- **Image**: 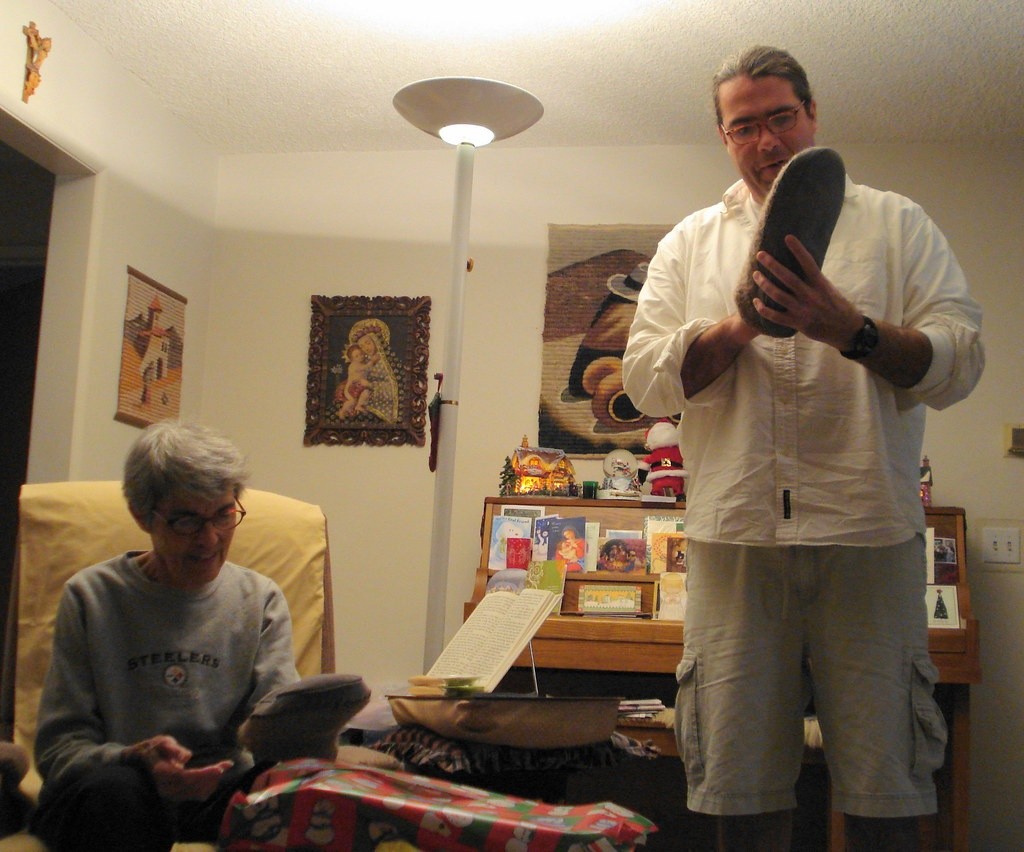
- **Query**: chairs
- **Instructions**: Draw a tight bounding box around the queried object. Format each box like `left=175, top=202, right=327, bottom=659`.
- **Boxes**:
left=0, top=482, right=334, bottom=851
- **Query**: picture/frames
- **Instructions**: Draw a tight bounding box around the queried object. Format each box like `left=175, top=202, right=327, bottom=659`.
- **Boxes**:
left=304, top=296, right=432, bottom=448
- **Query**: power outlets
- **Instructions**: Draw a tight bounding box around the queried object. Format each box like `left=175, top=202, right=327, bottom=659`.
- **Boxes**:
left=983, top=526, right=1019, bottom=563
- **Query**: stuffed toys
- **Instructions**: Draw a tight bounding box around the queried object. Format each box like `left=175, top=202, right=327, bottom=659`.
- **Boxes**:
left=638, top=418, right=688, bottom=501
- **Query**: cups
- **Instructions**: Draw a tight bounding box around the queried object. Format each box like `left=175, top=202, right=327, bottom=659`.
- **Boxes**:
left=583, top=481, right=598, bottom=499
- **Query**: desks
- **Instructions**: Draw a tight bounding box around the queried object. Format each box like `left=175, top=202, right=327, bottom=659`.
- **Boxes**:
left=371, top=694, right=626, bottom=807
left=465, top=498, right=980, bottom=851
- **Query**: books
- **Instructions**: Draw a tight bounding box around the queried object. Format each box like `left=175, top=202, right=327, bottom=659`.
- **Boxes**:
left=423, top=588, right=564, bottom=694
left=488, top=503, right=648, bottom=575
left=640, top=516, right=691, bottom=620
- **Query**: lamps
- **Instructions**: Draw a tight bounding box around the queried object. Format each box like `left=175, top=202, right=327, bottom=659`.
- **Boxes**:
left=393, top=77, right=544, bottom=678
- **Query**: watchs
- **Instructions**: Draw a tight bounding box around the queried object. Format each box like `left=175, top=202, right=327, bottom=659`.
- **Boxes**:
left=840, top=315, right=880, bottom=360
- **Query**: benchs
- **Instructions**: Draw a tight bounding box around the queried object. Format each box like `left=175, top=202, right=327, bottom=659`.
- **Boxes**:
left=617, top=709, right=847, bottom=852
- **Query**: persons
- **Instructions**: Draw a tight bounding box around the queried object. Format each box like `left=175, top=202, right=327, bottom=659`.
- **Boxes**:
left=622, top=41, right=988, bottom=852
left=32, top=422, right=302, bottom=852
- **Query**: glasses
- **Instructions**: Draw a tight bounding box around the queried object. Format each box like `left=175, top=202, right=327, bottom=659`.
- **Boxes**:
left=718, top=99, right=805, bottom=145
left=146, top=492, right=245, bottom=534
